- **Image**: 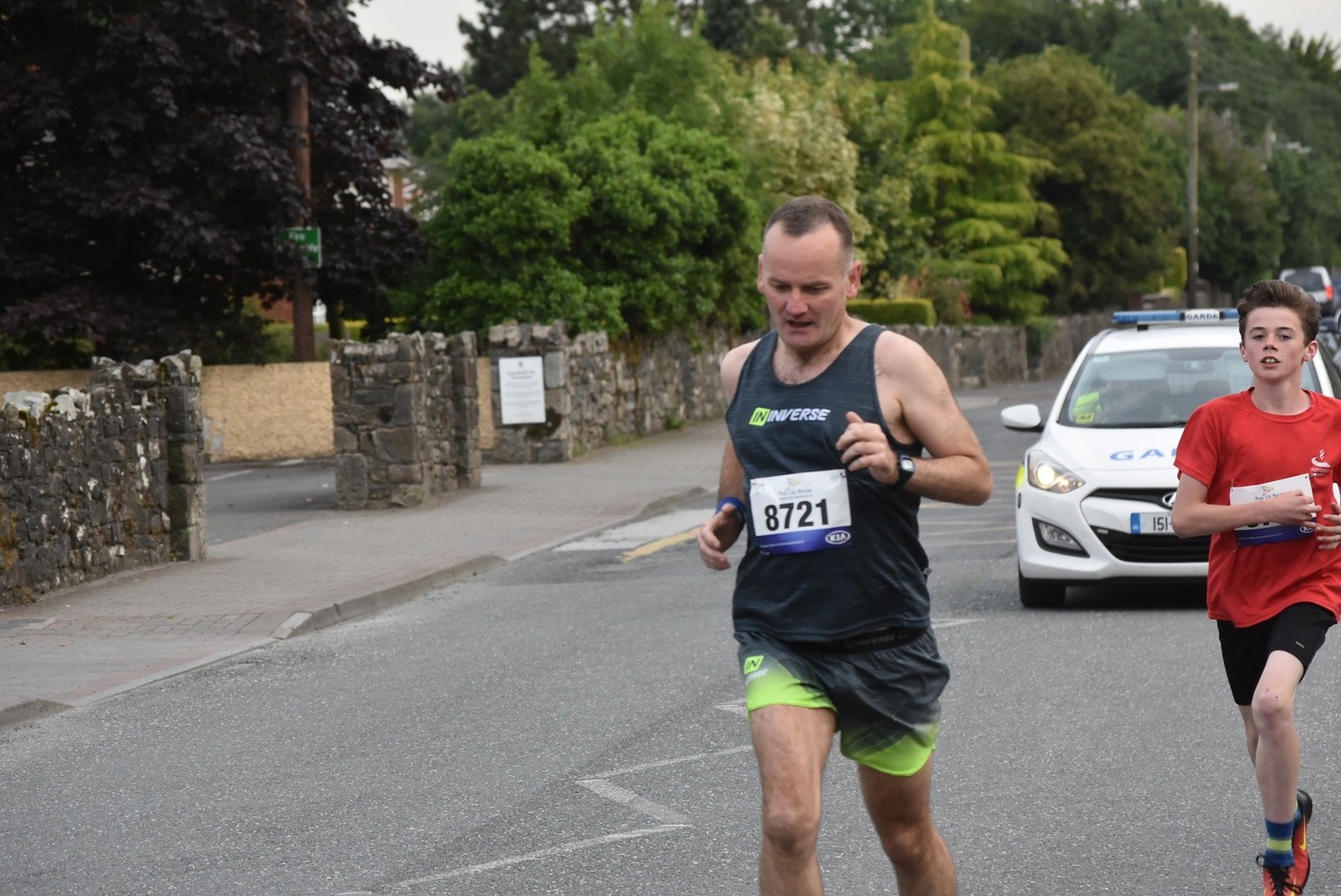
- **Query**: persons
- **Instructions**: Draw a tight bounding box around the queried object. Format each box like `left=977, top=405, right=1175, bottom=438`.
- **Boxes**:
left=696, top=194, right=991, bottom=895
left=1172, top=279, right=1341, bottom=896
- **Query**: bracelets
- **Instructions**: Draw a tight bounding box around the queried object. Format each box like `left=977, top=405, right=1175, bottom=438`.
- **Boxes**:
left=714, top=496, right=746, bottom=524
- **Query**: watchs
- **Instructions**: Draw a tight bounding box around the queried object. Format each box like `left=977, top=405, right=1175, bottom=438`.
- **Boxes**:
left=894, top=453, right=915, bottom=488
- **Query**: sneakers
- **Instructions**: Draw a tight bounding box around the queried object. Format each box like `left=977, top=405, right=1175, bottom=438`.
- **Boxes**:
left=1255, top=854, right=1297, bottom=896
left=1291, top=790, right=1313, bottom=891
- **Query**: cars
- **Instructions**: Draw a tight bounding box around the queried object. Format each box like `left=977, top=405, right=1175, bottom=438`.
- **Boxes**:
left=1280, top=265, right=1337, bottom=314
left=998, top=308, right=1340, bottom=610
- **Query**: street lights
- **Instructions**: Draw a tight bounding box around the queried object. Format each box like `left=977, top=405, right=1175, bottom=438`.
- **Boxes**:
left=1185, top=82, right=1238, bottom=306
